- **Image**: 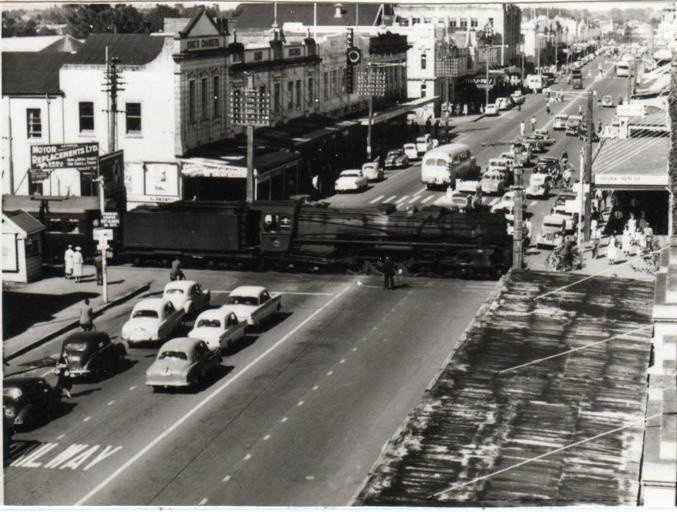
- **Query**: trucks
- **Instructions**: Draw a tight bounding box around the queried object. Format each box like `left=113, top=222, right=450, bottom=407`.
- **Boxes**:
left=571, top=69, right=582, bottom=88
left=445, top=179, right=482, bottom=208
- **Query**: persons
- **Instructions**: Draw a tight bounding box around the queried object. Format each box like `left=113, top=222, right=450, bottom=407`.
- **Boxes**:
left=94, top=252, right=105, bottom=284
left=63, top=244, right=75, bottom=280
left=519, top=82, right=659, bottom=273
left=78, top=298, right=98, bottom=332
left=70, top=246, right=85, bottom=283
left=2, top=343, right=10, bottom=379
left=382, top=256, right=397, bottom=291
left=398, top=115, right=449, bottom=144
left=55, top=350, right=74, bottom=398
left=169, top=257, right=185, bottom=281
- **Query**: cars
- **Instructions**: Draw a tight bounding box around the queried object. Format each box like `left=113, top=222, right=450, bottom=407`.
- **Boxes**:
left=334, top=162, right=384, bottom=193
left=3, top=376, right=63, bottom=430
left=481, top=129, right=560, bottom=222
left=56, top=330, right=126, bottom=383
left=536, top=190, right=578, bottom=248
left=602, top=94, right=613, bottom=107
left=188, top=308, right=247, bottom=352
left=482, top=90, right=524, bottom=115
left=163, top=280, right=209, bottom=314
left=220, top=285, right=281, bottom=326
left=553, top=114, right=599, bottom=142
left=146, top=337, right=222, bottom=393
left=616, top=62, right=630, bottom=78
left=385, top=136, right=433, bottom=168
left=121, top=298, right=184, bottom=348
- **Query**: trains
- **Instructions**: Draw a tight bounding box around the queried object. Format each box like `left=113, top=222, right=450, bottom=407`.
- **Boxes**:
left=2, top=193, right=512, bottom=282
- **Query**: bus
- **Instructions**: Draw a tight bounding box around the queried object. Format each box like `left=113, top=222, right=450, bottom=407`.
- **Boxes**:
left=421, top=143, right=476, bottom=189
